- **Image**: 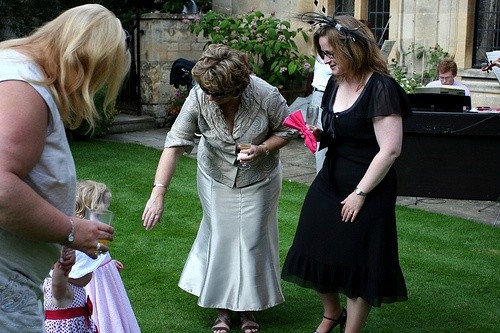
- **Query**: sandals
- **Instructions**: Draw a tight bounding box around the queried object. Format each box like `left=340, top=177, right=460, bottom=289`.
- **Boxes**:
left=239, top=310, right=261, bottom=333
left=212, top=316, right=232, bottom=333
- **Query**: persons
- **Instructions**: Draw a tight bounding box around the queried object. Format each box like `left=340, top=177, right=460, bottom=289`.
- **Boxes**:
left=312, top=20, right=372, bottom=173
left=142, top=43, right=299, bottom=333
left=495, top=58, right=500, bottom=68
left=281, top=6, right=413, bottom=333
left=0, top=4, right=143, bottom=333
left=424, top=59, right=472, bottom=107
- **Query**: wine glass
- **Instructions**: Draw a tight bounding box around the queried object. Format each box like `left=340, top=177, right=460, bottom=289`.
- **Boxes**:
left=237, top=126, right=253, bottom=171
left=303, top=106, right=319, bottom=146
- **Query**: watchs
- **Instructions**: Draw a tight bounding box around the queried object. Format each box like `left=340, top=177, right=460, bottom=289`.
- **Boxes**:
left=353, top=186, right=367, bottom=196
left=59, top=216, right=75, bottom=245
left=262, top=143, right=270, bottom=156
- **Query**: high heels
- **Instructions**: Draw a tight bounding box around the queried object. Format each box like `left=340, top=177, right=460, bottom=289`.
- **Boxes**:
left=313, top=307, right=347, bottom=333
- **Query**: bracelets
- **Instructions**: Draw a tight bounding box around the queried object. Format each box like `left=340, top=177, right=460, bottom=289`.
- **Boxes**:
left=153, top=184, right=168, bottom=190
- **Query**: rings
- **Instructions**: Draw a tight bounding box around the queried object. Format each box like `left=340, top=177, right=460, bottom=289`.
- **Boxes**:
left=96, top=242, right=101, bottom=250
left=349, top=211, right=352, bottom=214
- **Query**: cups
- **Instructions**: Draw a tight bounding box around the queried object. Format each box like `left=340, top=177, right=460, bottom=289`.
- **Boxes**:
left=85, top=209, right=114, bottom=256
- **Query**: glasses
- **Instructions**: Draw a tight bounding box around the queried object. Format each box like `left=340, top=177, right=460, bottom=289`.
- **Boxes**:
left=198, top=82, right=233, bottom=102
left=319, top=47, right=343, bottom=60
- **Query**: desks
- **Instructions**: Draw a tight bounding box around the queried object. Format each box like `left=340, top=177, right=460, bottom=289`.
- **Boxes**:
left=396, top=109, right=500, bottom=202
